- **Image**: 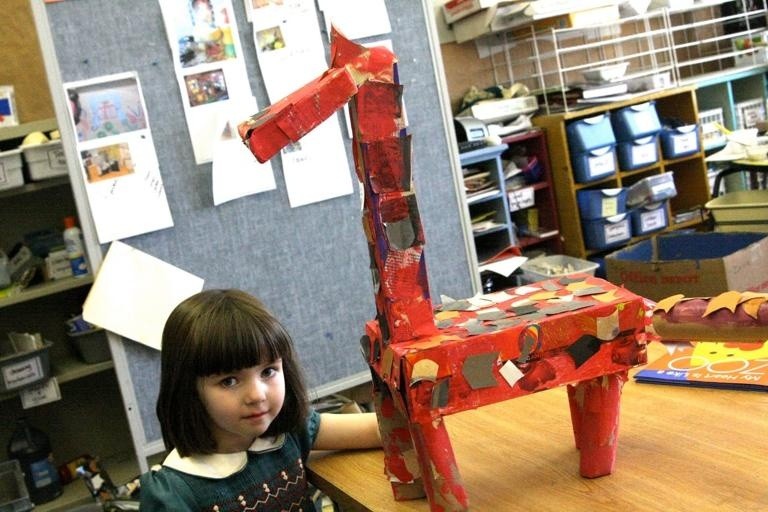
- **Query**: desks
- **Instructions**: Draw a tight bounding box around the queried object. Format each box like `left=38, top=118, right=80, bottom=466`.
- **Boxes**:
left=307, top=337, right=767, bottom=512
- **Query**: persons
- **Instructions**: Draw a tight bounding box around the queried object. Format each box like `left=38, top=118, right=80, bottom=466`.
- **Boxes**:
left=140, top=289, right=386, bottom=511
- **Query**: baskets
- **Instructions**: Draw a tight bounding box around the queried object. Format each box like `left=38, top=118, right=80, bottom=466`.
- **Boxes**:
left=698, top=107, right=726, bottom=147
left=734, top=99, right=764, bottom=129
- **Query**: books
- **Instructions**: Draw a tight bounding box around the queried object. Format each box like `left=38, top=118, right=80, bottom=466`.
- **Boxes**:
left=634, top=340, right=768, bottom=392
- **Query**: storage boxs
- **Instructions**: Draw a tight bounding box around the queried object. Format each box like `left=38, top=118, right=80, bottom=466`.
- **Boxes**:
left=0, top=148, right=24, bottom=192
left=603, top=232, right=767, bottom=301
left=0, top=339, right=53, bottom=397
left=19, top=138, right=68, bottom=181
left=67, top=328, right=112, bottom=364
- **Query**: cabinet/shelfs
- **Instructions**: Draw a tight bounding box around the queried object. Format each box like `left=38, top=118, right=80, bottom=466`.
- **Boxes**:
left=0, top=116, right=142, bottom=512
